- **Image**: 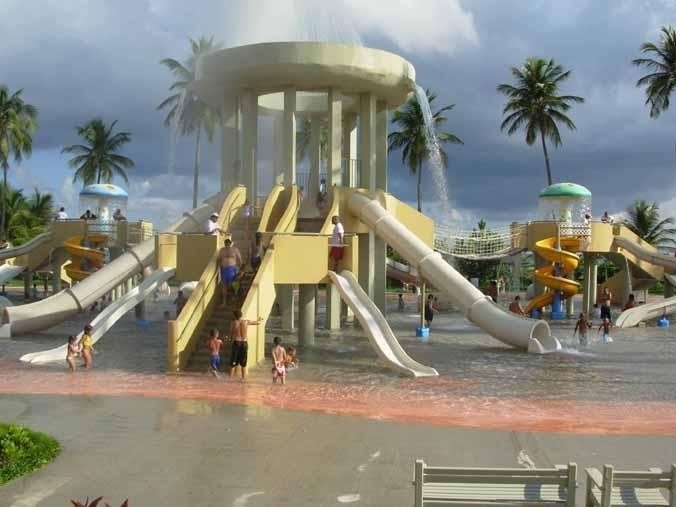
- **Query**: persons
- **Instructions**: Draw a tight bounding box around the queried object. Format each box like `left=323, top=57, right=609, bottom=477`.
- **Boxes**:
left=572, top=310, right=595, bottom=348
left=433, top=296, right=442, bottom=312
left=595, top=316, right=616, bottom=344
left=296, top=183, right=304, bottom=209
left=200, top=209, right=231, bottom=236
left=250, top=229, right=269, bottom=272
left=327, top=213, right=345, bottom=272
left=227, top=309, right=264, bottom=380
left=113, top=208, right=128, bottom=220
left=398, top=292, right=406, bottom=312
left=0, top=238, right=9, bottom=250
left=625, top=292, right=637, bottom=309
left=171, top=289, right=188, bottom=317
left=509, top=294, right=525, bottom=315
left=285, top=346, right=298, bottom=370
left=481, top=278, right=498, bottom=303
left=29, top=282, right=42, bottom=300
left=601, top=211, right=615, bottom=223
left=78, top=209, right=97, bottom=219
left=314, top=174, right=337, bottom=221
left=202, top=326, right=227, bottom=382
left=237, top=199, right=255, bottom=232
left=54, top=206, right=68, bottom=219
left=425, top=293, right=441, bottom=327
left=269, top=335, right=290, bottom=385
left=598, top=285, right=617, bottom=328
left=218, top=238, right=242, bottom=307
left=78, top=324, right=97, bottom=367
left=65, top=333, right=81, bottom=372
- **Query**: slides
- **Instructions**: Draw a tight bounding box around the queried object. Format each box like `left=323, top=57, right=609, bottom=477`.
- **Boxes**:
left=348, top=193, right=562, bottom=354
left=20, top=268, right=177, bottom=363
left=0, top=194, right=224, bottom=338
left=523, top=236, right=582, bottom=313
left=329, top=269, right=440, bottom=378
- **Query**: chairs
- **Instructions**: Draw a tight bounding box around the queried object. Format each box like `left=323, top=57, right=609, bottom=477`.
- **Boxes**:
left=584, top=463, right=676, bottom=506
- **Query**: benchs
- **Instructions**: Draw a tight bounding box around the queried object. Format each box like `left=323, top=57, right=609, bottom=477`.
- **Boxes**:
left=411, top=457, right=580, bottom=506
